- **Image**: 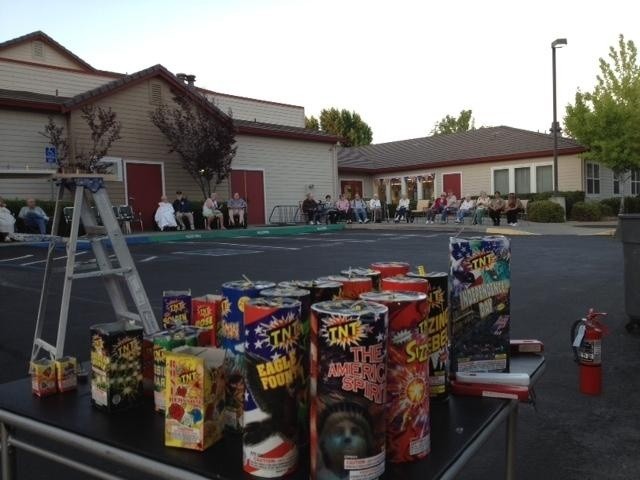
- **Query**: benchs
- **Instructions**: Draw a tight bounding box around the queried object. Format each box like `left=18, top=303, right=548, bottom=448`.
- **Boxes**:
left=64, top=206, right=143, bottom=234
left=299, top=201, right=390, bottom=223
left=446, top=200, right=528, bottom=226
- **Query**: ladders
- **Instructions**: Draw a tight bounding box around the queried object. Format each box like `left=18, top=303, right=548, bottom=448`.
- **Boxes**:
left=28, top=174, right=161, bottom=375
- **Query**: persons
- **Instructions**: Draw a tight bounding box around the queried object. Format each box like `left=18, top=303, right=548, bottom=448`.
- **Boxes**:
left=0, top=199, right=16, bottom=242
left=173, top=191, right=195, bottom=231
left=18, top=198, right=50, bottom=234
left=202, top=193, right=227, bottom=230
left=302, top=190, right=525, bottom=227
left=154, top=196, right=177, bottom=231
left=227, top=192, right=247, bottom=226
left=316, top=401, right=374, bottom=480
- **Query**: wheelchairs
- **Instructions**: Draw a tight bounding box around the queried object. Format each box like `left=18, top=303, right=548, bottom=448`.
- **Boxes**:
left=395, top=203, right=414, bottom=224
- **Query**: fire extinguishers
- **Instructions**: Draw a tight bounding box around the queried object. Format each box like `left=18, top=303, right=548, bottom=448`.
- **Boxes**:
left=570, top=308, right=610, bottom=395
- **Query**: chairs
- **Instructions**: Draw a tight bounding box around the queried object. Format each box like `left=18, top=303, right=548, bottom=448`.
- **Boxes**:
left=204, top=216, right=220, bottom=229
left=411, top=199, right=431, bottom=223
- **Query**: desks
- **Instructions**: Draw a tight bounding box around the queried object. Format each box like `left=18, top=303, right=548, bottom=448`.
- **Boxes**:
left=0, top=352, right=546, bottom=480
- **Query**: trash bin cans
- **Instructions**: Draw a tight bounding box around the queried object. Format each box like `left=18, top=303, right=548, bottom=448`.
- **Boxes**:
left=617, top=213, right=640, bottom=334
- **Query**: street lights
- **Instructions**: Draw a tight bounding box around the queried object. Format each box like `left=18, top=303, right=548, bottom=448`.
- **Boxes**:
left=549, top=36, right=568, bottom=194
